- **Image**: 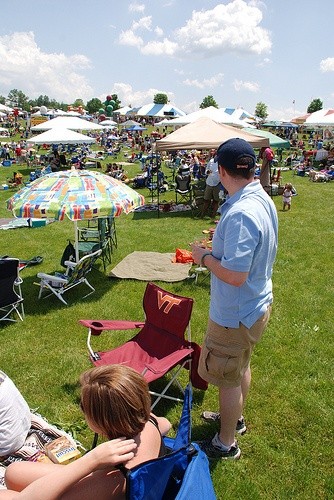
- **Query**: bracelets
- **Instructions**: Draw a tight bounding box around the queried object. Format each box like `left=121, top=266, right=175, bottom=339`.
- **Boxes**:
left=201, top=253, right=211, bottom=264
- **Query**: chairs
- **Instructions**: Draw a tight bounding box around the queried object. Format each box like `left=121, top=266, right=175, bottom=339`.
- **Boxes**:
left=0, top=215, right=217, bottom=500
left=146, top=166, right=195, bottom=204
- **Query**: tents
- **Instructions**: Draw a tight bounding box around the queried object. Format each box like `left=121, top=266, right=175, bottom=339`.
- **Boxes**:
left=26, top=127, right=98, bottom=169
left=99, top=103, right=257, bottom=131
left=31, top=116, right=114, bottom=130
left=152, top=119, right=290, bottom=217
left=0, top=104, right=81, bottom=121
left=261, top=108, right=334, bottom=131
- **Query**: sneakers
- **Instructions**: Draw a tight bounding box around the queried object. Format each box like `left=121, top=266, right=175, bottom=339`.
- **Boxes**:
left=202, top=411, right=246, bottom=434
left=192, top=432, right=241, bottom=461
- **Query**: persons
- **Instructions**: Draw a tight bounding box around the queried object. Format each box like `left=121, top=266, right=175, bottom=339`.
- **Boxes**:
left=190, top=138, right=278, bottom=466
left=0, top=363, right=171, bottom=500
left=191, top=147, right=292, bottom=220
left=0, top=116, right=195, bottom=192
left=277, top=126, right=334, bottom=179
left=12, top=436, right=138, bottom=500
left=0, top=370, right=31, bottom=462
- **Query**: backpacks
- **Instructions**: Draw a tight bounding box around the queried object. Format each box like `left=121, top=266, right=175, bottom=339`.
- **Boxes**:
left=61, top=241, right=76, bottom=268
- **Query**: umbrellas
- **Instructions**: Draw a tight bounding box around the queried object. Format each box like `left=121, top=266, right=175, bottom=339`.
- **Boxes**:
left=7, top=166, right=144, bottom=263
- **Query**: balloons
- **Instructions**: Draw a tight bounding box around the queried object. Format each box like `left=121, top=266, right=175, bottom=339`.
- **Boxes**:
left=99, top=94, right=120, bottom=121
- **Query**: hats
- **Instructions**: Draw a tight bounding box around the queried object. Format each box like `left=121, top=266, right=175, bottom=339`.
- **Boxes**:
left=212, top=137, right=255, bottom=173
